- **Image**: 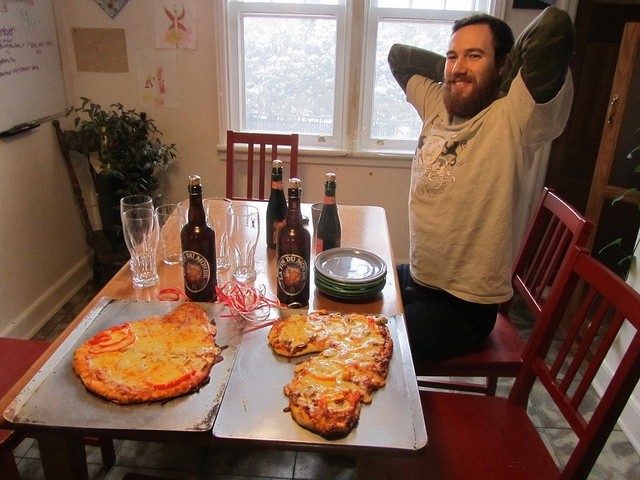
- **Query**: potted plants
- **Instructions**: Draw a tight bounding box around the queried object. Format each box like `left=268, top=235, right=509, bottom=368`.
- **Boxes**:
left=66, top=99, right=175, bottom=249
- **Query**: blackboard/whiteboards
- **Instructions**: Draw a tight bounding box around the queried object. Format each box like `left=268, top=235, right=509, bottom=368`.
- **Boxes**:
left=1, top=0, right=70, bottom=138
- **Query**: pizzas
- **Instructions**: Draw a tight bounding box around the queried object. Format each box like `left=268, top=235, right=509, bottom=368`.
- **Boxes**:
left=267, top=309, right=394, bottom=440
left=72, top=302, right=229, bottom=406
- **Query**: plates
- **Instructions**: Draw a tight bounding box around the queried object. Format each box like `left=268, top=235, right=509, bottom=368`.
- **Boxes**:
left=313, top=247, right=387, bottom=299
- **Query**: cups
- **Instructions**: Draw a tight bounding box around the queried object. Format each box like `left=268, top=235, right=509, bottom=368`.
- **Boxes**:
left=227, top=205, right=260, bottom=281
left=122, top=208, right=160, bottom=288
left=177, top=199, right=209, bottom=233
left=121, top=195, right=154, bottom=271
left=156, top=204, right=188, bottom=265
left=202, top=197, right=234, bottom=273
left=311, top=202, right=338, bottom=233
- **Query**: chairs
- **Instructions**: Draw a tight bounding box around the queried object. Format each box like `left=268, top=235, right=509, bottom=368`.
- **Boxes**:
left=52, top=120, right=131, bottom=288
left=356, top=249, right=640, bottom=480
left=228, top=130, right=297, bottom=201
left=414, top=185, right=594, bottom=397
left=0, top=338, right=117, bottom=480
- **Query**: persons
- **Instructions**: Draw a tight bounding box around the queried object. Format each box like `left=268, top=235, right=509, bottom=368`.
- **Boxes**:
left=388, top=9, right=573, bottom=367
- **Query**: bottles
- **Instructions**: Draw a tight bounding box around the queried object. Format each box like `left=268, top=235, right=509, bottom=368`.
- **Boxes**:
left=277, top=178, right=310, bottom=307
left=181, top=175, right=217, bottom=302
left=267, top=160, right=288, bottom=250
left=316, top=173, right=340, bottom=255
